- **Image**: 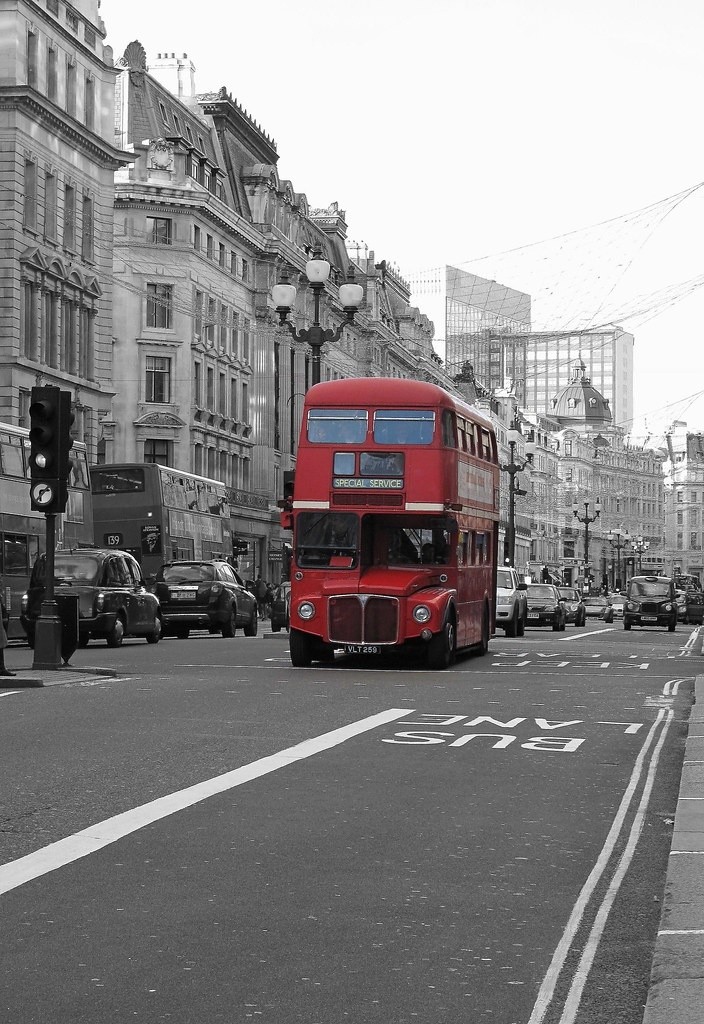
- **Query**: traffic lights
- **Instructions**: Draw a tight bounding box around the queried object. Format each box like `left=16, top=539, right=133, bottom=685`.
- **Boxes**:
left=29, top=387, right=61, bottom=512
left=283, top=470, right=294, bottom=529
left=232, top=536, right=248, bottom=568
left=60, top=391, right=75, bottom=513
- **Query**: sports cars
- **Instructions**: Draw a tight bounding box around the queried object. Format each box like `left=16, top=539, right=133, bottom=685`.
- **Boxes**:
left=583, top=597, right=614, bottom=623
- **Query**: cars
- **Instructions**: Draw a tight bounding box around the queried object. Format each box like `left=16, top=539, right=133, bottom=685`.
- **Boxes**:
left=557, top=586, right=587, bottom=627
left=675, top=591, right=691, bottom=623
left=621, top=575, right=681, bottom=631
left=526, top=583, right=567, bottom=631
left=610, top=596, right=626, bottom=617
left=23, top=548, right=165, bottom=648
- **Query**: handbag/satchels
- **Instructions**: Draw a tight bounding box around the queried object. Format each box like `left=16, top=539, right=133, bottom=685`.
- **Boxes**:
left=258, top=583, right=268, bottom=598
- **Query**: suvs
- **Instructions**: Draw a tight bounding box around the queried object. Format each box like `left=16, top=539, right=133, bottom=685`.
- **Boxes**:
left=495, top=567, right=528, bottom=637
left=270, top=582, right=292, bottom=629
left=152, top=559, right=259, bottom=638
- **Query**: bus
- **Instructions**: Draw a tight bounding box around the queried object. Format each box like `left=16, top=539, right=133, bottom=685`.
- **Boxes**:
left=88, top=463, right=234, bottom=592
left=0, top=422, right=95, bottom=640
left=278, top=376, right=498, bottom=665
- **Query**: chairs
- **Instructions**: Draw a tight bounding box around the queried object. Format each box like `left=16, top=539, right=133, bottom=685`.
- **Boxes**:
left=421, top=543, right=435, bottom=564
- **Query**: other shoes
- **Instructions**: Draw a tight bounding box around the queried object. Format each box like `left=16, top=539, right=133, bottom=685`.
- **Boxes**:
left=0, top=670, right=16, bottom=676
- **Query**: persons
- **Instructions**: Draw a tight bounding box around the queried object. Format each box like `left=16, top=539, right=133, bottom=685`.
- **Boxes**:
left=330, top=521, right=356, bottom=556
left=419, top=543, right=444, bottom=564
left=256, top=576, right=279, bottom=621
left=0, top=605, right=15, bottom=676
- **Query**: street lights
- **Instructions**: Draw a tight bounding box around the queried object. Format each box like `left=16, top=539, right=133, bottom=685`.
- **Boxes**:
left=267, top=243, right=366, bottom=386
left=631, top=534, right=651, bottom=575
left=606, top=523, right=631, bottom=592
left=573, top=491, right=601, bottom=596
left=498, top=420, right=537, bottom=567
